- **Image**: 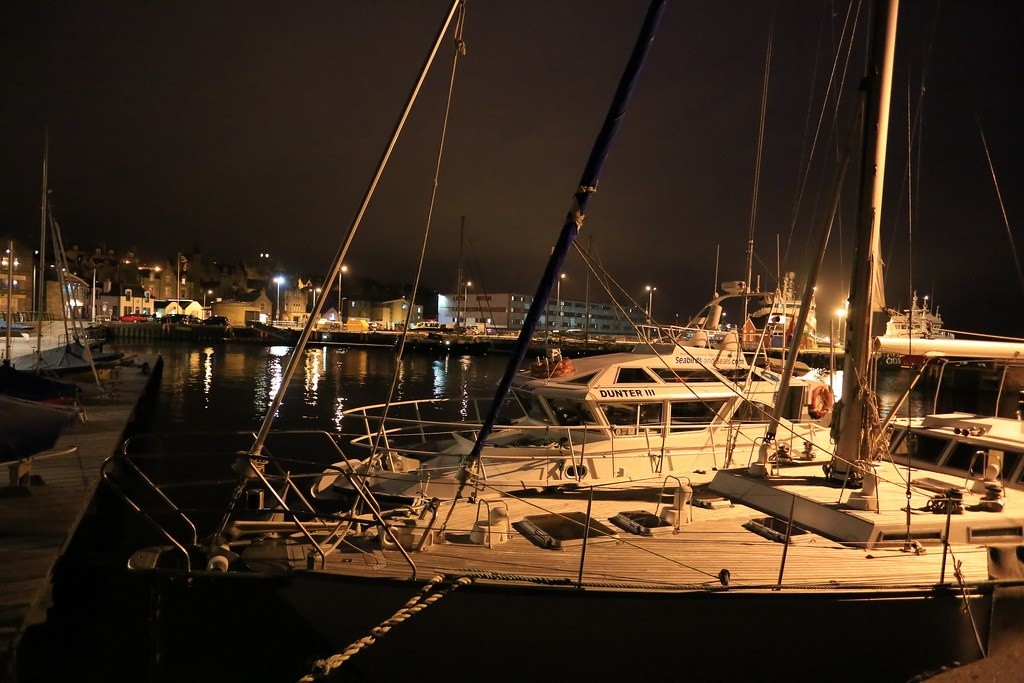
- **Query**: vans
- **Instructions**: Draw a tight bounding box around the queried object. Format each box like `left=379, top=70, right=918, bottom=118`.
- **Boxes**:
left=412, top=322, right=437, bottom=333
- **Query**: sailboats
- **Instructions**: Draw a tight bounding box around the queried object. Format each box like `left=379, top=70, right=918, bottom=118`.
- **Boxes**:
left=0, top=134, right=123, bottom=395
left=96, top=1, right=1023, bottom=659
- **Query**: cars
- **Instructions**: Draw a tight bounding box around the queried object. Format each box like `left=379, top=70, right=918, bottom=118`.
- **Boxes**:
left=486, top=329, right=502, bottom=336
left=201, top=316, right=230, bottom=327
left=118, top=314, right=147, bottom=324
left=157, top=314, right=189, bottom=324
left=472, top=329, right=481, bottom=335
left=187, top=314, right=203, bottom=324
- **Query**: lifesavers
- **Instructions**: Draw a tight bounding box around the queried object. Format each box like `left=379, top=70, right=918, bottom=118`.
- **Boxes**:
left=530, top=355, right=571, bottom=378
left=807, top=387, right=833, bottom=419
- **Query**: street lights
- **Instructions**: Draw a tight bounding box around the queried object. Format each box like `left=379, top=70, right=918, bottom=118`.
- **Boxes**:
left=313, top=288, right=321, bottom=307
left=338, top=266, right=347, bottom=315
left=276, top=277, right=284, bottom=321
left=464, top=281, right=472, bottom=327
left=557, top=273, right=566, bottom=320
left=646, top=285, right=657, bottom=343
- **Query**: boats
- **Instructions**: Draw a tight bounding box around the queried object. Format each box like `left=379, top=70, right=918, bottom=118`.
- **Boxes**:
left=0, top=397, right=78, bottom=468
left=881, top=291, right=954, bottom=369
